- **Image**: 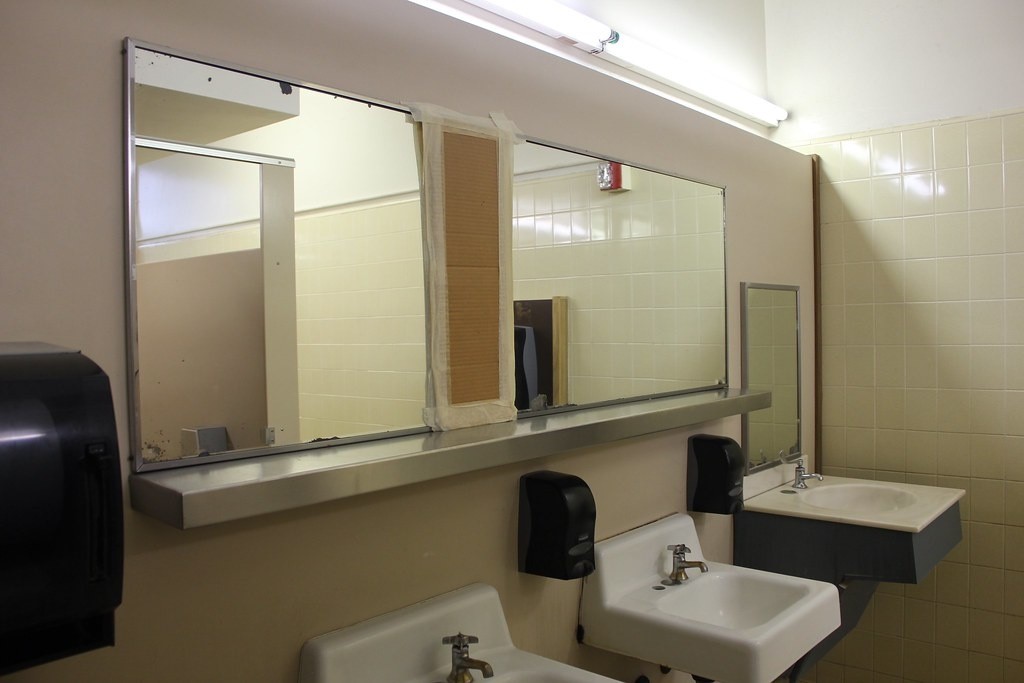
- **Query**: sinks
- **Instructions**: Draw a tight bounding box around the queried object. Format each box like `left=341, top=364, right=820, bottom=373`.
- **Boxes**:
left=296, top=582, right=620, bottom=683
left=803, top=485, right=916, bottom=513
left=579, top=513, right=842, bottom=683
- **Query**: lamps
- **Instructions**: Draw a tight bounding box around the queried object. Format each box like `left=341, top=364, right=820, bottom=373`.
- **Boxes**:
left=595, top=29, right=790, bottom=129
left=461, top=0, right=616, bottom=55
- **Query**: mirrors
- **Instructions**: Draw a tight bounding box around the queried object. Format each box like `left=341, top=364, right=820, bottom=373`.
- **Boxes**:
left=120, top=33, right=436, bottom=475
left=510, top=131, right=731, bottom=427
left=739, top=278, right=804, bottom=477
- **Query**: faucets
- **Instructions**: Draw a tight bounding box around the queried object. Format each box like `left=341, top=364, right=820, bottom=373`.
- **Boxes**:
left=666, top=543, right=708, bottom=579
left=758, top=448, right=767, bottom=464
left=440, top=633, right=494, bottom=683
left=779, top=452, right=824, bottom=489
